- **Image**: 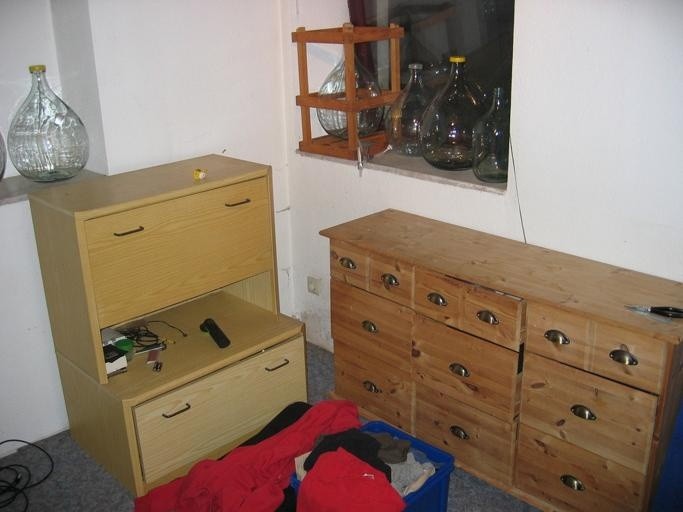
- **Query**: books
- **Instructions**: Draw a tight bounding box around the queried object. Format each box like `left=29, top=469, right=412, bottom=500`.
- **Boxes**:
left=107, top=367, right=127, bottom=379
left=102, top=344, right=127, bottom=373
left=100, top=327, right=124, bottom=347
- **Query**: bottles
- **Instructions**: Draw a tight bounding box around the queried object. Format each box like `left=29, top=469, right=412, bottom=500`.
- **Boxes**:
left=316, top=46, right=383, bottom=141
left=6, top=65, right=89, bottom=183
left=384, top=55, right=508, bottom=184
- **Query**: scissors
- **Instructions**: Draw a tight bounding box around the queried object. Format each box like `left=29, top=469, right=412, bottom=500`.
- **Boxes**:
left=624, top=305, right=683, bottom=318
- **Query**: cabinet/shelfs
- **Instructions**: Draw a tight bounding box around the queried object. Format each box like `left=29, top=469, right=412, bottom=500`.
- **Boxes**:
left=513, top=301, right=681, bottom=510
left=411, top=263, right=517, bottom=493
left=330, top=239, right=413, bottom=442
left=24, top=154, right=277, bottom=384
left=50, top=326, right=308, bottom=495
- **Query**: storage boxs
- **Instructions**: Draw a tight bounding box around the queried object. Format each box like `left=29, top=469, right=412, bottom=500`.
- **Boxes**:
left=289, top=422, right=455, bottom=510
